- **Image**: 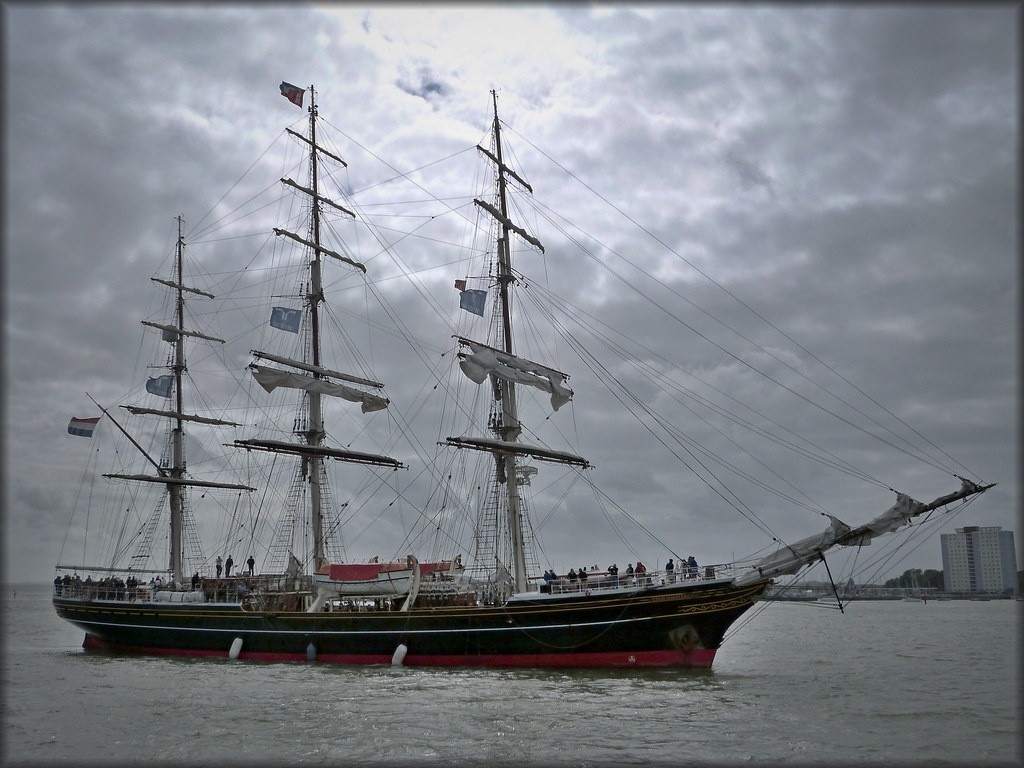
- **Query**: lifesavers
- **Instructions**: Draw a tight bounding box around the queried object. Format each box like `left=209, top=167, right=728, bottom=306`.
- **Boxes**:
left=585, top=590, right=590, bottom=597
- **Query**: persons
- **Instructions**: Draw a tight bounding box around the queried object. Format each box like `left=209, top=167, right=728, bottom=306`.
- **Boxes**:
left=216, top=556, right=223, bottom=578
left=54, top=575, right=139, bottom=603
left=225, top=555, right=233, bottom=578
left=247, top=555, right=255, bottom=577
left=367, top=598, right=381, bottom=611
left=543, top=555, right=700, bottom=591
left=192, top=572, right=199, bottom=591
left=149, top=575, right=166, bottom=587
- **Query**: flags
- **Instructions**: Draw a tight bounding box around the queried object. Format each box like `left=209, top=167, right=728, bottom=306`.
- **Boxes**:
left=454, top=279, right=488, bottom=317
left=279, top=80, right=306, bottom=109
left=146, top=375, right=174, bottom=397
left=269, top=306, right=303, bottom=334
left=68, top=416, right=100, bottom=438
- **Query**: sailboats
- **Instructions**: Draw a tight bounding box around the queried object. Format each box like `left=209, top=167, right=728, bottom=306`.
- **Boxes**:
left=51, top=77, right=998, bottom=669
